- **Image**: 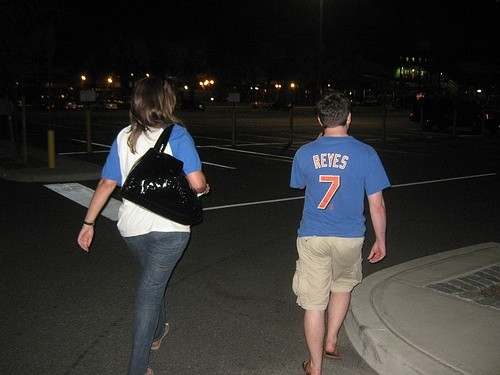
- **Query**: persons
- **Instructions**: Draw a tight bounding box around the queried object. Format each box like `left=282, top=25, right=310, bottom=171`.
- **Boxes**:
left=290, top=93, right=390, bottom=375
left=78, top=75, right=209, bottom=375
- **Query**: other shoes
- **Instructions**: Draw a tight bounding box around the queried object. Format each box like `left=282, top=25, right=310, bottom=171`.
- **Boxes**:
left=151, top=322, right=169, bottom=349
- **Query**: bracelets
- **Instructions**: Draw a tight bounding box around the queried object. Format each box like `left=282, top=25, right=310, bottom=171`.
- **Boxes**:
left=83, top=221, right=94, bottom=225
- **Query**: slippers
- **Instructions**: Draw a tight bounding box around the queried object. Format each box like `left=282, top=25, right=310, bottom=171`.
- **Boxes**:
left=323, top=349, right=340, bottom=359
left=303, top=360, right=311, bottom=375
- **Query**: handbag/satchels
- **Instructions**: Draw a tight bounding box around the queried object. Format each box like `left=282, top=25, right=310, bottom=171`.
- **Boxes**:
left=120, top=125, right=203, bottom=225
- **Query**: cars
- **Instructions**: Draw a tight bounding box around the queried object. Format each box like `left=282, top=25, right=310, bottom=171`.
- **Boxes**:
left=410, top=93, right=500, bottom=132
left=36, top=90, right=120, bottom=112
